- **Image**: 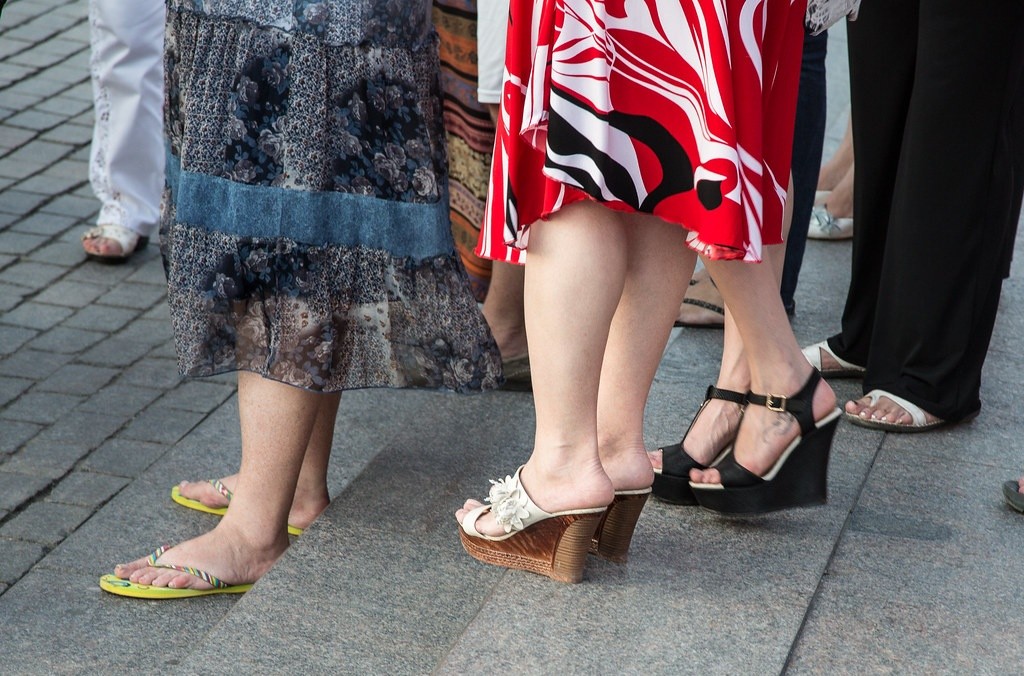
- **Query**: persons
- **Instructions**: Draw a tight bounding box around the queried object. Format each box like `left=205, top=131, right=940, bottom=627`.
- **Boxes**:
left=454, top=0, right=808, bottom=584
left=671, top=169, right=794, bottom=328
left=98, top=0, right=505, bottom=599
left=81, top=0, right=165, bottom=264
left=646, top=167, right=844, bottom=516
left=780, top=0, right=862, bottom=319
left=801, top=0, right=1023, bottom=512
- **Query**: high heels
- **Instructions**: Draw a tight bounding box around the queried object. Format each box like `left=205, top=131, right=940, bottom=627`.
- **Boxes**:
left=588, top=486, right=652, bottom=562
left=688, top=366, right=843, bottom=516
left=650, top=384, right=749, bottom=505
left=459, top=464, right=608, bottom=583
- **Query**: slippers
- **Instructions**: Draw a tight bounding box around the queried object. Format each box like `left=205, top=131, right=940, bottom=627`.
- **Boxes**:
left=99, top=545, right=254, bottom=599
left=171, top=479, right=304, bottom=535
left=496, top=352, right=533, bottom=393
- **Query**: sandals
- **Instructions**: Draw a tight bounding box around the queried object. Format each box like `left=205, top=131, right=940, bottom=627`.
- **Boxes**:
left=845, top=389, right=981, bottom=432
left=673, top=276, right=795, bottom=330
left=81, top=224, right=148, bottom=266
left=801, top=340, right=866, bottom=380
left=1002, top=481, right=1024, bottom=513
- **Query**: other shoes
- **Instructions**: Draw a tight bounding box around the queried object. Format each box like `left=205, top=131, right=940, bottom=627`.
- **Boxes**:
left=807, top=205, right=853, bottom=239
left=814, top=190, right=832, bottom=205
